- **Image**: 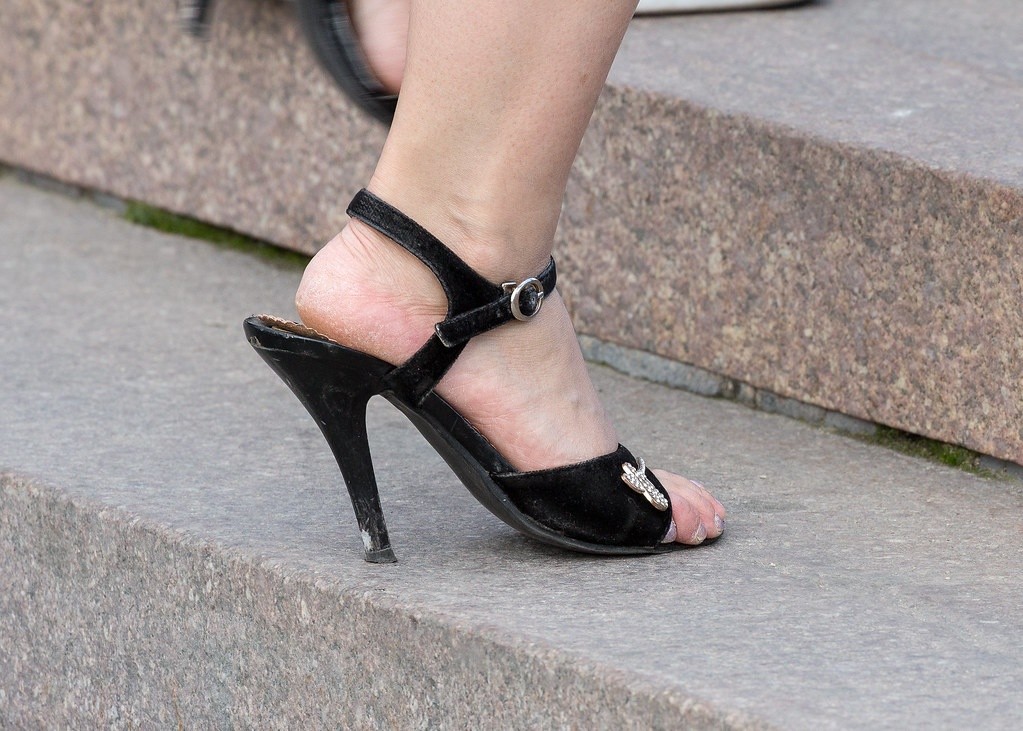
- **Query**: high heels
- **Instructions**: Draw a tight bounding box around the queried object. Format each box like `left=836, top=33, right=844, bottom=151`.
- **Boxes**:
left=187, top=0, right=401, bottom=127
left=243, top=190, right=720, bottom=566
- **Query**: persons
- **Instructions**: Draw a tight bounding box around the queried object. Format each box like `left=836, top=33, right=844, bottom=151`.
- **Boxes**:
left=241, top=0, right=726, bottom=566
